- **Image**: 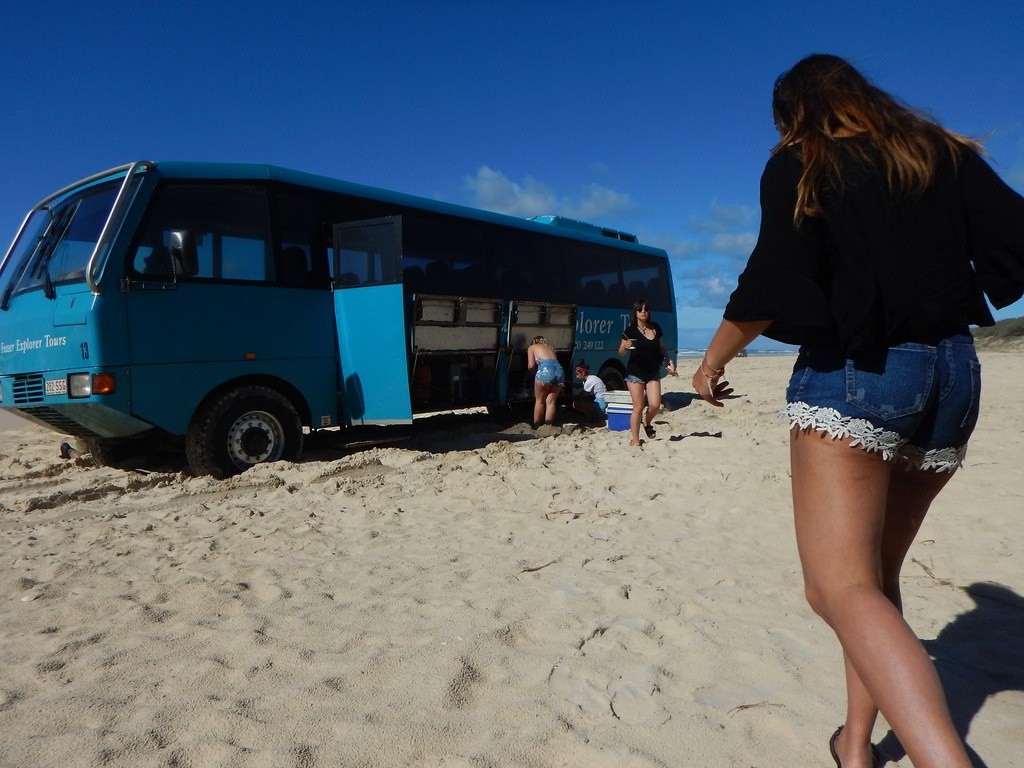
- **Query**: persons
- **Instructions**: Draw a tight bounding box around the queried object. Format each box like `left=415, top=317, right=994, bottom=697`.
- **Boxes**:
left=618, top=299, right=675, bottom=446
left=692, top=55, right=1024, bottom=768
left=570, top=363, right=607, bottom=424
left=528, top=335, right=565, bottom=424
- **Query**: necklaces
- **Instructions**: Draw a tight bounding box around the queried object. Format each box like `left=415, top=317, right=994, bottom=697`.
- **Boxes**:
left=638, top=324, right=649, bottom=334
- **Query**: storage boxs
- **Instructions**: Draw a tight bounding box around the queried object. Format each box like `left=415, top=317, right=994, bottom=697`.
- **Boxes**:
left=606, top=404, right=634, bottom=432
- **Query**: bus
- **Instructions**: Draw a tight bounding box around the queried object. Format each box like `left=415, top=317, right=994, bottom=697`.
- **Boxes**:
left=1, top=158, right=678, bottom=481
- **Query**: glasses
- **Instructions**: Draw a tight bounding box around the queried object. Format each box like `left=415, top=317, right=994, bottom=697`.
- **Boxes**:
left=635, top=307, right=649, bottom=313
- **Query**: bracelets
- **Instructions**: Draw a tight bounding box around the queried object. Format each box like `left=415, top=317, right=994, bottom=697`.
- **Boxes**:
left=700, top=351, right=725, bottom=398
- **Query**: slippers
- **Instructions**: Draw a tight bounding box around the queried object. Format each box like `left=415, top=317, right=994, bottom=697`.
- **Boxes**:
left=830, top=725, right=877, bottom=768
left=643, top=424, right=656, bottom=439
